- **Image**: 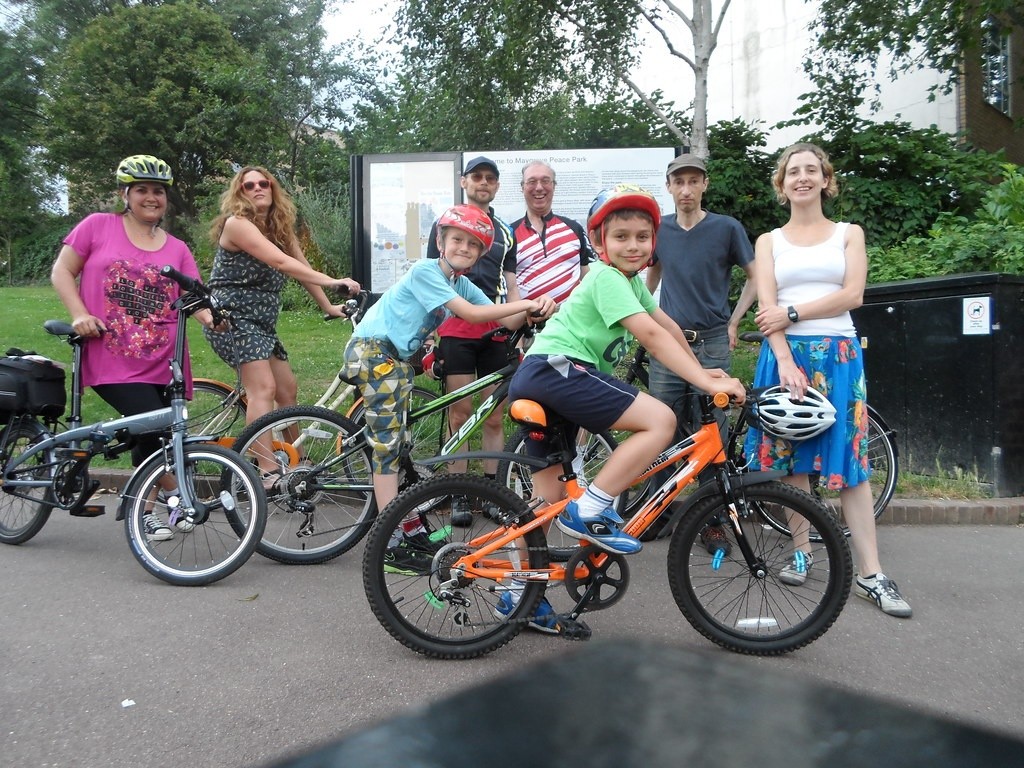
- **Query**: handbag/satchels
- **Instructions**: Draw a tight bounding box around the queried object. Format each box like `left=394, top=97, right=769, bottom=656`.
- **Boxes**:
left=0, top=348, right=67, bottom=414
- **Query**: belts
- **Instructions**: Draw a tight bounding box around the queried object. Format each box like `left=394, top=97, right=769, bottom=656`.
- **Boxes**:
left=681, top=325, right=727, bottom=343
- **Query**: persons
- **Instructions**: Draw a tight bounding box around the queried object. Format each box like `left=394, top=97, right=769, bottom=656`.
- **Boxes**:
left=429, top=157, right=517, bottom=526
left=50, top=153, right=230, bottom=541
left=342, top=204, right=556, bottom=576
left=507, top=161, right=591, bottom=489
left=203, top=167, right=360, bottom=495
left=493, top=185, right=746, bottom=634
left=639, top=152, right=756, bottom=556
left=742, top=142, right=913, bottom=619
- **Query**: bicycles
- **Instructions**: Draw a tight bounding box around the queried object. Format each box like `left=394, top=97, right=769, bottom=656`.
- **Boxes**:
left=188, top=284, right=899, bottom=658
left=0, top=264, right=278, bottom=587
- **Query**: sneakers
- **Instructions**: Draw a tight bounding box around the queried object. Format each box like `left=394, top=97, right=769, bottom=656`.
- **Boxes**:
left=383, top=541, right=434, bottom=575
left=494, top=591, right=560, bottom=634
left=555, top=500, right=643, bottom=554
left=142, top=509, right=174, bottom=540
left=167, top=494, right=193, bottom=532
left=855, top=573, right=912, bottom=617
left=398, top=531, right=448, bottom=557
left=640, top=515, right=672, bottom=541
left=450, top=495, right=472, bottom=526
left=482, top=500, right=501, bottom=523
left=702, top=523, right=731, bottom=556
left=780, top=553, right=814, bottom=584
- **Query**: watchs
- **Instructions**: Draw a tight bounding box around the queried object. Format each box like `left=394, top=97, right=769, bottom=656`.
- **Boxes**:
left=788, top=304, right=799, bottom=327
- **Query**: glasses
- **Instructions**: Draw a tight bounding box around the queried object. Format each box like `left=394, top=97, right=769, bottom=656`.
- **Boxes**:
left=464, top=173, right=498, bottom=185
left=523, top=179, right=554, bottom=187
left=241, top=180, right=271, bottom=191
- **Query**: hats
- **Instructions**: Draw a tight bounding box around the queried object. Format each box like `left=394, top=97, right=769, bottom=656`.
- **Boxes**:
left=666, top=154, right=707, bottom=177
left=464, top=156, right=498, bottom=179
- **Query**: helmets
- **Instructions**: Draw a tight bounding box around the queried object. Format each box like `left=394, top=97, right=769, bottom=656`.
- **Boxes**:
left=756, top=385, right=837, bottom=441
left=117, top=155, right=173, bottom=187
left=436, top=204, right=496, bottom=258
left=587, top=184, right=660, bottom=263
